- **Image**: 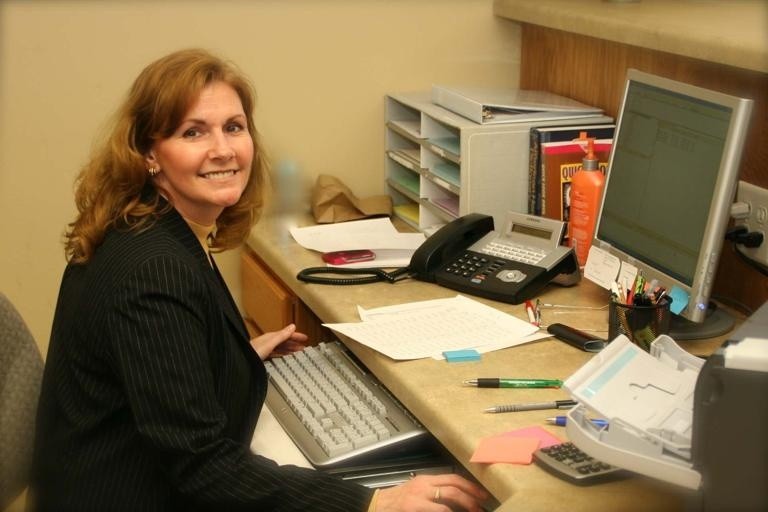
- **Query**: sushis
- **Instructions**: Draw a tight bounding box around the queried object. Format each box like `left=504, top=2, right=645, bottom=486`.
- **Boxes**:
left=531, top=442, right=639, bottom=486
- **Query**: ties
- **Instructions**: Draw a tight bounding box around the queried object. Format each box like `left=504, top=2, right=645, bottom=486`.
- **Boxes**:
left=582, top=68, right=754, bottom=340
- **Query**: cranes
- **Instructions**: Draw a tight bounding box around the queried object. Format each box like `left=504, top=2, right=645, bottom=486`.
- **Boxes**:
left=431, top=85, right=605, bottom=124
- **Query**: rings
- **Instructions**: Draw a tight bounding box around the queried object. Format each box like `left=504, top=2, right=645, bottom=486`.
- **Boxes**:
left=485, top=400, right=578, bottom=413
left=462, top=378, right=564, bottom=389
left=525, top=299, right=541, bottom=327
left=611, top=268, right=667, bottom=355
left=544, top=416, right=609, bottom=428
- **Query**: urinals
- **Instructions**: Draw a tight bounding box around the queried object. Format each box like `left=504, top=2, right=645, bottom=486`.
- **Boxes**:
left=442, top=349, right=481, bottom=363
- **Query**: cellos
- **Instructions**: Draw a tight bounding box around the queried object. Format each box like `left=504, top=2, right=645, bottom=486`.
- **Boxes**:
left=563, top=298, right=768, bottom=512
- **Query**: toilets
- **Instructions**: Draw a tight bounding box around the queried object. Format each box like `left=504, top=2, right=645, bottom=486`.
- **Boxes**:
left=409, top=209, right=582, bottom=305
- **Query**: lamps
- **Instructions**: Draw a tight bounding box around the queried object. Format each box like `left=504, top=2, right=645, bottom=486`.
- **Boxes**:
left=0, top=290, right=46, bottom=511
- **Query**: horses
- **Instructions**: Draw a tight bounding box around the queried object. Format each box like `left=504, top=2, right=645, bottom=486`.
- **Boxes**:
left=322, top=247, right=376, bottom=265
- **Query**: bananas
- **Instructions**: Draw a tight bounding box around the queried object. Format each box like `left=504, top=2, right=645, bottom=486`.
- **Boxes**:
left=262, top=340, right=431, bottom=470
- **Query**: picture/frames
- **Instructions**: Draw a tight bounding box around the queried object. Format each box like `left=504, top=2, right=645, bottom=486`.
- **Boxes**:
left=240, top=203, right=753, bottom=512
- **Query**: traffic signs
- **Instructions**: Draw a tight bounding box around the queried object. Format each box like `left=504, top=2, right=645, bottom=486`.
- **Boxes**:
left=732, top=180, right=768, bottom=269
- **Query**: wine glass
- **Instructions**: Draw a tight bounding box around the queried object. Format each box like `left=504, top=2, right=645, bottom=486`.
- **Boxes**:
left=433, top=486, right=440, bottom=503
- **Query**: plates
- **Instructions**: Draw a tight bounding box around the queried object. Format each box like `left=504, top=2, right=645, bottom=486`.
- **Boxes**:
left=380, top=92, right=616, bottom=241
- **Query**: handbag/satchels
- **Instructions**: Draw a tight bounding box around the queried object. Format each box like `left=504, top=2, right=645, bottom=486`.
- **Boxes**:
left=368, top=488, right=380, bottom=512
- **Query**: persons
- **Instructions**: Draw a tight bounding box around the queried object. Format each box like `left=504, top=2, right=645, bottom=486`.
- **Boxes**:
left=28, top=47, right=489, bottom=512
